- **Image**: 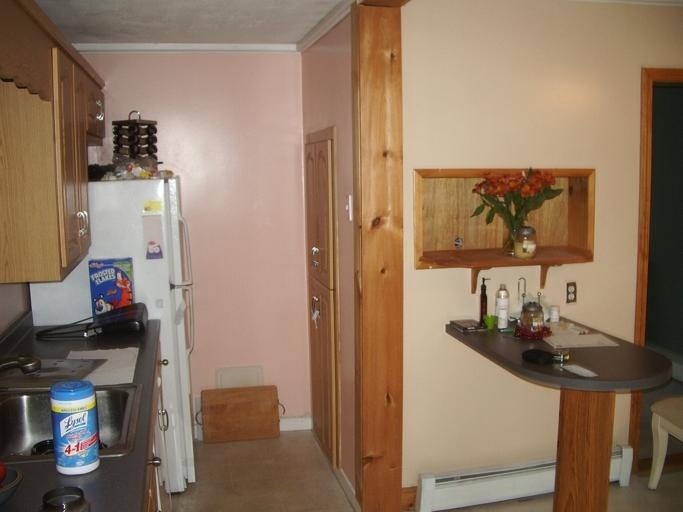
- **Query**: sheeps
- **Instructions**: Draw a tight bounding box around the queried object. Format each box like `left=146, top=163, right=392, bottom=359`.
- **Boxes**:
left=1, top=356, right=42, bottom=375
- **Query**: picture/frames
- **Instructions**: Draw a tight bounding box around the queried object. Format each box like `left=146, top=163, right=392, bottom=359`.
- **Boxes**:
left=445, top=316, right=673, bottom=512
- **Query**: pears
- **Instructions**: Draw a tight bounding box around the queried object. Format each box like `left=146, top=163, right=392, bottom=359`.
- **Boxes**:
left=495, top=281, right=510, bottom=331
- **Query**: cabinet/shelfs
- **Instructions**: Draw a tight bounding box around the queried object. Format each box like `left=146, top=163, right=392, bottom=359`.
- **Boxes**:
left=47, top=379, right=101, bottom=478
left=518, top=302, right=545, bottom=342
left=513, top=226, right=537, bottom=259
left=549, top=304, right=561, bottom=323
left=494, top=283, right=510, bottom=330
left=37, top=484, right=91, bottom=512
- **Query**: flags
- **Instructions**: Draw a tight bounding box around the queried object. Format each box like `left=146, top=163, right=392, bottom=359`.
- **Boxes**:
left=502, top=214, right=533, bottom=259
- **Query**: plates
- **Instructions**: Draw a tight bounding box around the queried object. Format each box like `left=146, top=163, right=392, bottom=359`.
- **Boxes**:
left=1, top=51, right=105, bottom=286
left=143, top=341, right=173, bottom=512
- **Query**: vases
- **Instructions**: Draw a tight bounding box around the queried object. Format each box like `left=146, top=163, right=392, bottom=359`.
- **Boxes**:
left=470, top=168, right=565, bottom=251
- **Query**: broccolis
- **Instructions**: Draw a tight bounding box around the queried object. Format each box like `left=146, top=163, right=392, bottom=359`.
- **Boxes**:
left=26, top=174, right=197, bottom=494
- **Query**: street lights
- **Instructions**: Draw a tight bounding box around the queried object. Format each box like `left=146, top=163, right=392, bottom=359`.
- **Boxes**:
left=86, top=302, right=148, bottom=337
